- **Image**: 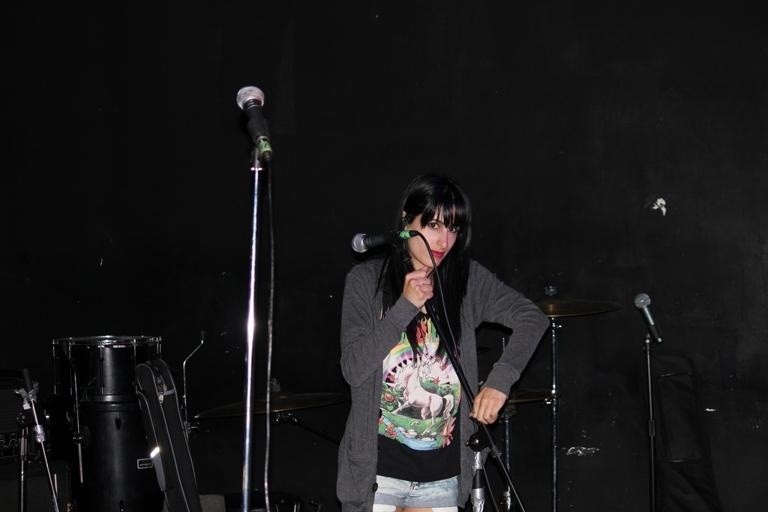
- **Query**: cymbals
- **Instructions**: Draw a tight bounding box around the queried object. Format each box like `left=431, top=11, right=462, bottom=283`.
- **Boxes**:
left=194, top=393, right=342, bottom=416
left=512, top=388, right=552, bottom=405
left=533, top=299, right=621, bottom=317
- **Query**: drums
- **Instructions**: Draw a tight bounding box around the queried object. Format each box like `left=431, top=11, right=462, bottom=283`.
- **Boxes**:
left=53, top=336, right=161, bottom=404
left=64, top=405, right=166, bottom=512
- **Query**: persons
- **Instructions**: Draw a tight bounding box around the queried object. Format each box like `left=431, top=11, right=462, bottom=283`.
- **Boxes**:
left=336, top=172, right=550, bottom=512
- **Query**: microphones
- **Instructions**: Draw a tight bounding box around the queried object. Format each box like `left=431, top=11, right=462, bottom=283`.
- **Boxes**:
left=236, top=85, right=272, bottom=162
left=351, top=230, right=418, bottom=253
left=23, top=367, right=31, bottom=393
left=634, top=293, right=664, bottom=342
